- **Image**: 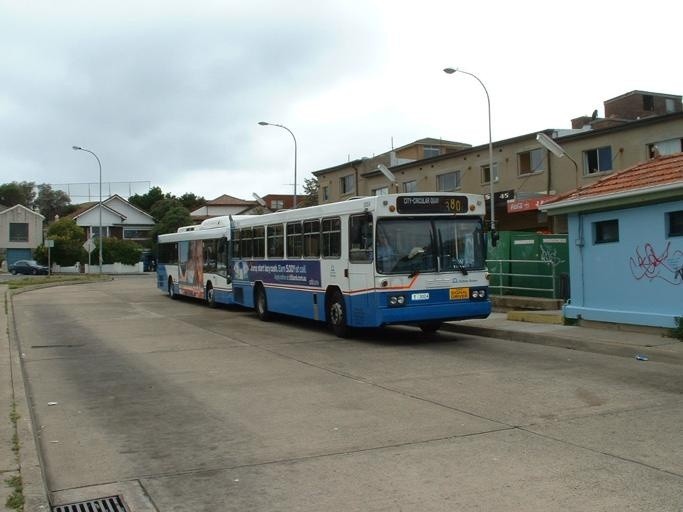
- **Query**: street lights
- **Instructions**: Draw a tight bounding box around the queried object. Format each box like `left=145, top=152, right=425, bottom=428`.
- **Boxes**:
left=71, top=146, right=102, bottom=274
left=256, top=120, right=299, bottom=208
left=442, top=66, right=498, bottom=232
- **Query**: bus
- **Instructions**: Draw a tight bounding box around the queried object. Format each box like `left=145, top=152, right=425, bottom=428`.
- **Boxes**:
left=228, top=191, right=502, bottom=338
left=156, top=211, right=264, bottom=308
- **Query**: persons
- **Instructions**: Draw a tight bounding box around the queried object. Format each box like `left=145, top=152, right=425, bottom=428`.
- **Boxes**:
left=408, top=237, right=425, bottom=259
left=374, top=233, right=394, bottom=259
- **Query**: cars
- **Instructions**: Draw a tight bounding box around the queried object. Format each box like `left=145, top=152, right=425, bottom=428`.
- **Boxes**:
left=7, top=260, right=52, bottom=275
